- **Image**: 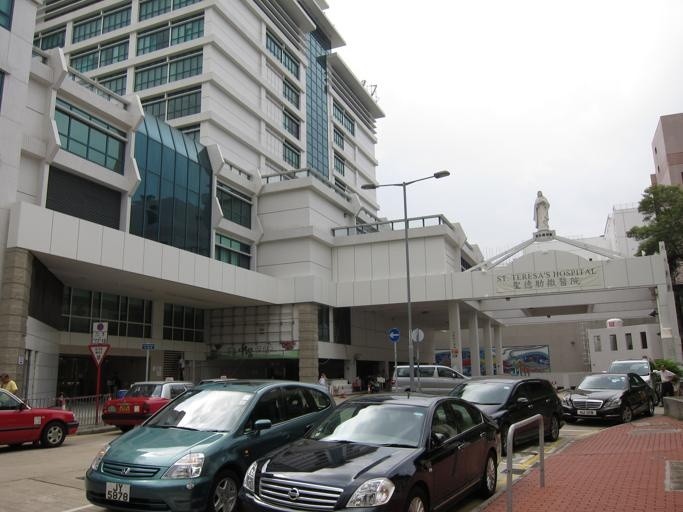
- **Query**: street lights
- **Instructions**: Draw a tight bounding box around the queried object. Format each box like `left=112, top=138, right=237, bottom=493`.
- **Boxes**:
left=361, top=169, right=451, bottom=392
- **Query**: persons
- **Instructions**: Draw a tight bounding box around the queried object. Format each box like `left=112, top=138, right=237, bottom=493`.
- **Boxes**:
left=653, top=363, right=679, bottom=407
left=0, top=371, right=18, bottom=395
left=534, top=189, right=550, bottom=229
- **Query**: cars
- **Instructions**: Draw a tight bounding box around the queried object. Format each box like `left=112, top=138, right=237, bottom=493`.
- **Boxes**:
left=0, top=387, right=80, bottom=448
